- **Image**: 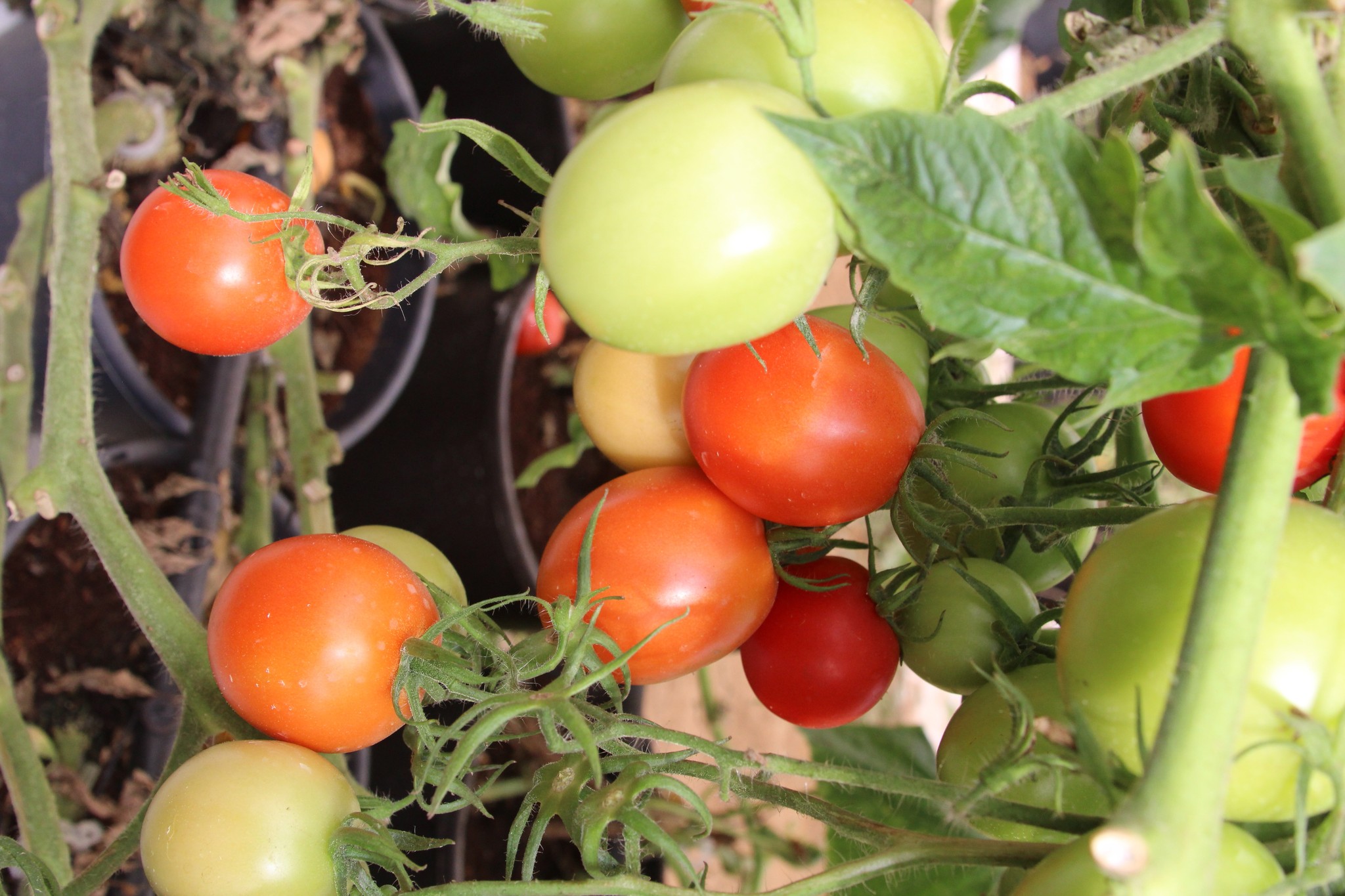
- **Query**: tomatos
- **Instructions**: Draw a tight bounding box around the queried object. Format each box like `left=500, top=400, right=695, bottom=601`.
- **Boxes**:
left=119, top=168, right=329, bottom=357
left=223, top=518, right=474, bottom=751
left=139, top=733, right=372, bottom=893
left=474, top=1, right=1345, bottom=891
